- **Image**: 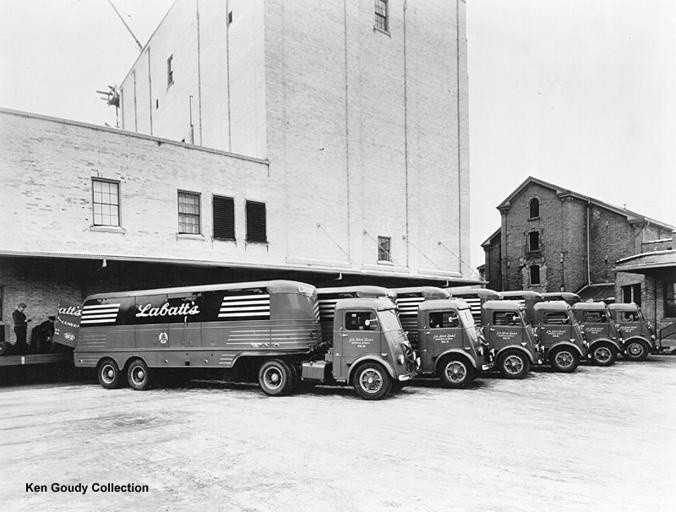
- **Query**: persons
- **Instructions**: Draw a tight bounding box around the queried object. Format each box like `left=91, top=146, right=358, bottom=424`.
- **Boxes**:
left=12, top=303, right=31, bottom=354
left=41, top=316, right=56, bottom=353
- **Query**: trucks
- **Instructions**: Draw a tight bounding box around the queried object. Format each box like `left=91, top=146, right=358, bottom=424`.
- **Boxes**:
left=313, top=285, right=497, bottom=390
left=71, top=280, right=422, bottom=399
left=440, top=285, right=548, bottom=381
left=500, top=291, right=592, bottom=374
left=539, top=291, right=626, bottom=369
left=584, top=296, right=658, bottom=361
